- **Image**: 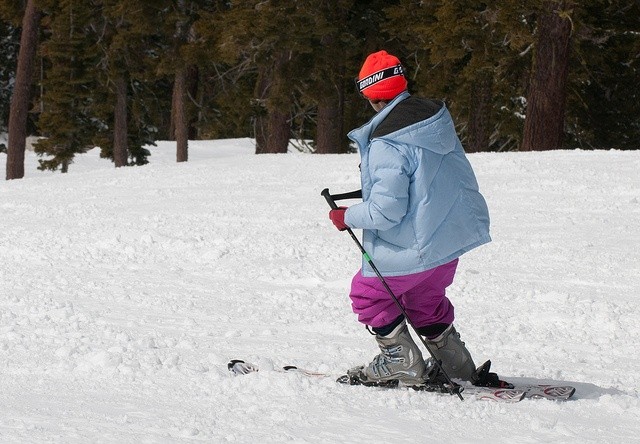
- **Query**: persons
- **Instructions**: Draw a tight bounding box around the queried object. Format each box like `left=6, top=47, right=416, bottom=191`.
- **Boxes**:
left=329, top=48, right=492, bottom=388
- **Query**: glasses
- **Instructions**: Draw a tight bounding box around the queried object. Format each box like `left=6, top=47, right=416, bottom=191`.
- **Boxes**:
left=354, top=64, right=405, bottom=96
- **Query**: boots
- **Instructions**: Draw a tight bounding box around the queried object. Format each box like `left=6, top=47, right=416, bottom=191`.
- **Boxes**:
left=365, top=325, right=426, bottom=381
left=424, top=324, right=476, bottom=380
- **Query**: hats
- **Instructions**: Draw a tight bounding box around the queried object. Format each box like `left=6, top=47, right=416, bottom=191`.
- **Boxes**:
left=358, top=50, right=408, bottom=100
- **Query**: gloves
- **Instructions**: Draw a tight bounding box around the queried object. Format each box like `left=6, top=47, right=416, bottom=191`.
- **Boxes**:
left=329, top=206, right=348, bottom=231
